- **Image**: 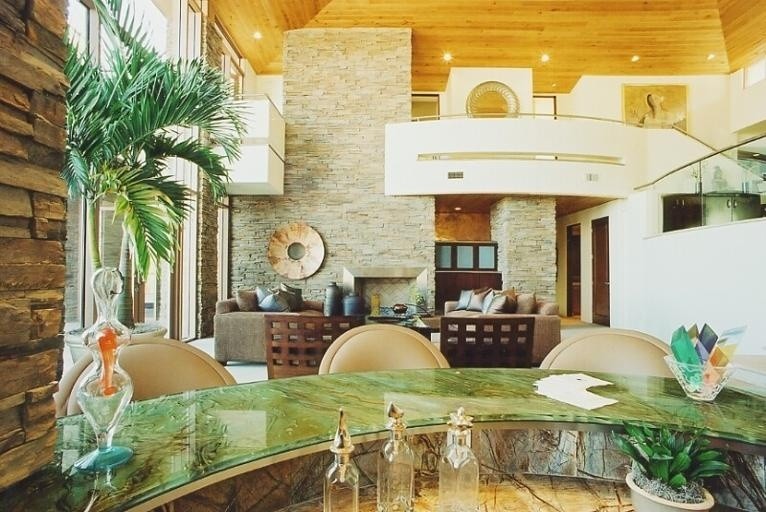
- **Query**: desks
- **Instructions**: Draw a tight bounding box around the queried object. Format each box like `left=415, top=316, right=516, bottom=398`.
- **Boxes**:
left=365, top=307, right=440, bottom=341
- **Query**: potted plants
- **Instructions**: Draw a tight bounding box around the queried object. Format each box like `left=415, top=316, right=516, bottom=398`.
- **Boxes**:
left=614, top=426, right=735, bottom=511
left=56, top=1, right=255, bottom=362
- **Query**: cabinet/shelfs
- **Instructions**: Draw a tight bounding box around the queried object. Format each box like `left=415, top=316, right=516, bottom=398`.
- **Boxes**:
left=435, top=272, right=503, bottom=310
left=664, top=194, right=704, bottom=232
left=705, top=192, right=761, bottom=227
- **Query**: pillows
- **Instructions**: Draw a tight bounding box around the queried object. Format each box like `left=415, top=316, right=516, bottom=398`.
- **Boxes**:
left=451, top=286, right=539, bottom=316
left=232, top=282, right=305, bottom=313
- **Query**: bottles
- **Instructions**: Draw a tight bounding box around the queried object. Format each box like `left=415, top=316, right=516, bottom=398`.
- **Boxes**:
left=441, top=405, right=479, bottom=512
left=370, top=291, right=381, bottom=315
left=324, top=405, right=360, bottom=511
left=372, top=404, right=419, bottom=511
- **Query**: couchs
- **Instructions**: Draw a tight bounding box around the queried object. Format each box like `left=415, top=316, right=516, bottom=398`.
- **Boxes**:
left=443, top=296, right=563, bottom=368
left=211, top=295, right=329, bottom=367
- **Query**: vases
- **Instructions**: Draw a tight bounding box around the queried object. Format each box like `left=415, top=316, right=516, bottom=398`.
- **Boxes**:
left=323, top=281, right=339, bottom=316
left=339, top=291, right=366, bottom=317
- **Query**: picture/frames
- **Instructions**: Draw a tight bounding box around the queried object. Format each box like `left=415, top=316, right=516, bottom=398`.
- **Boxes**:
left=621, top=81, right=691, bottom=135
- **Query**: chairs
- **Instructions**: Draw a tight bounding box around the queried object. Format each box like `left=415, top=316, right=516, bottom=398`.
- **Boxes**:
left=318, top=323, right=452, bottom=375
left=441, top=318, right=537, bottom=369
left=65, top=332, right=239, bottom=418
left=262, top=313, right=354, bottom=379
left=538, top=328, right=675, bottom=379
